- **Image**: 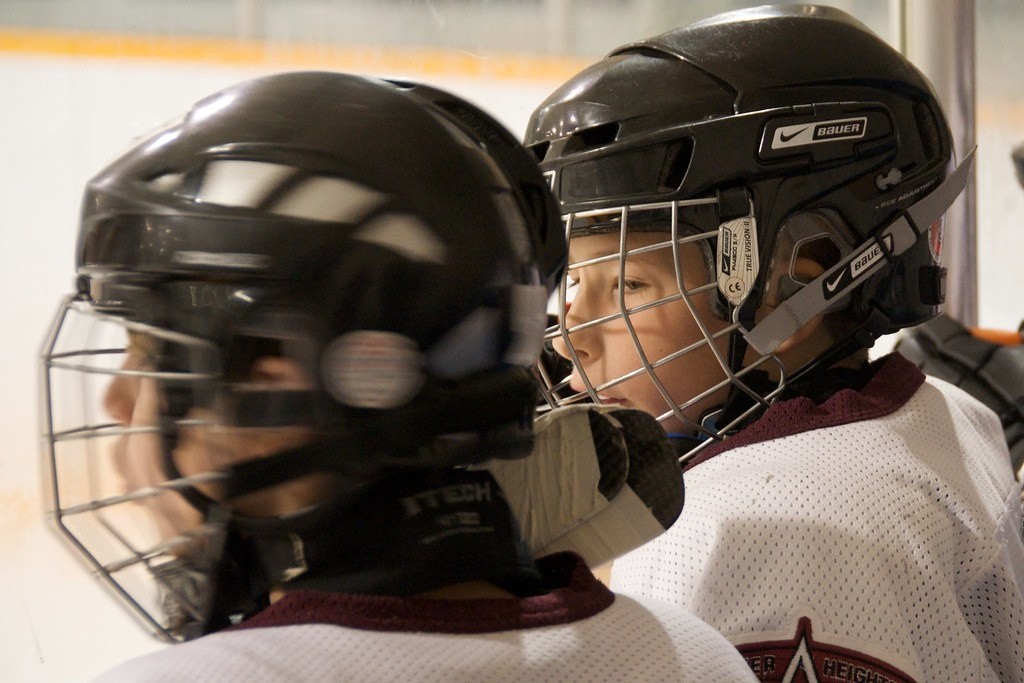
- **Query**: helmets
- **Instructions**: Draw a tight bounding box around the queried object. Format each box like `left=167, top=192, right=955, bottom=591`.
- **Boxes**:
left=525, top=2, right=955, bottom=468
left=34, top=67, right=572, bottom=645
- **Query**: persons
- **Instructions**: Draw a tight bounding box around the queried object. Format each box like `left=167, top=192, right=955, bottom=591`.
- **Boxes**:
left=42, top=70, right=761, bottom=683
left=523, top=8, right=1024, bottom=683
